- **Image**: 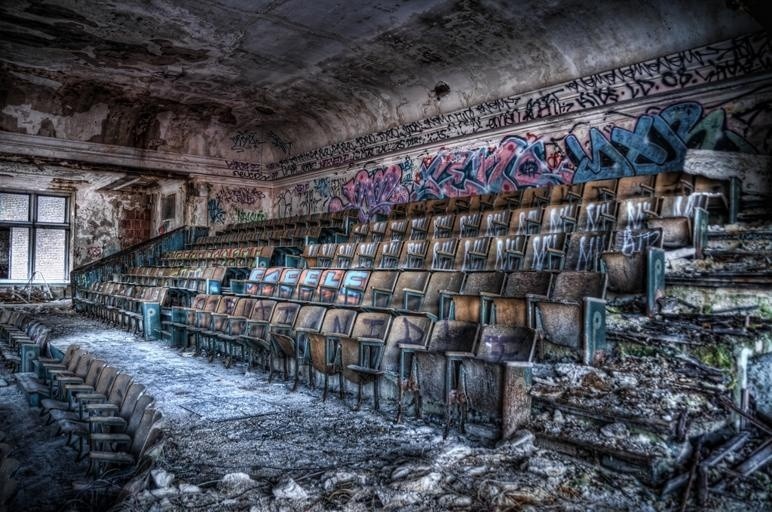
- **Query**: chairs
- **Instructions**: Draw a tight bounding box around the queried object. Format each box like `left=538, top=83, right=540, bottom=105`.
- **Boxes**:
left=74, top=170, right=743, bottom=445
left=0, top=305, right=165, bottom=512
